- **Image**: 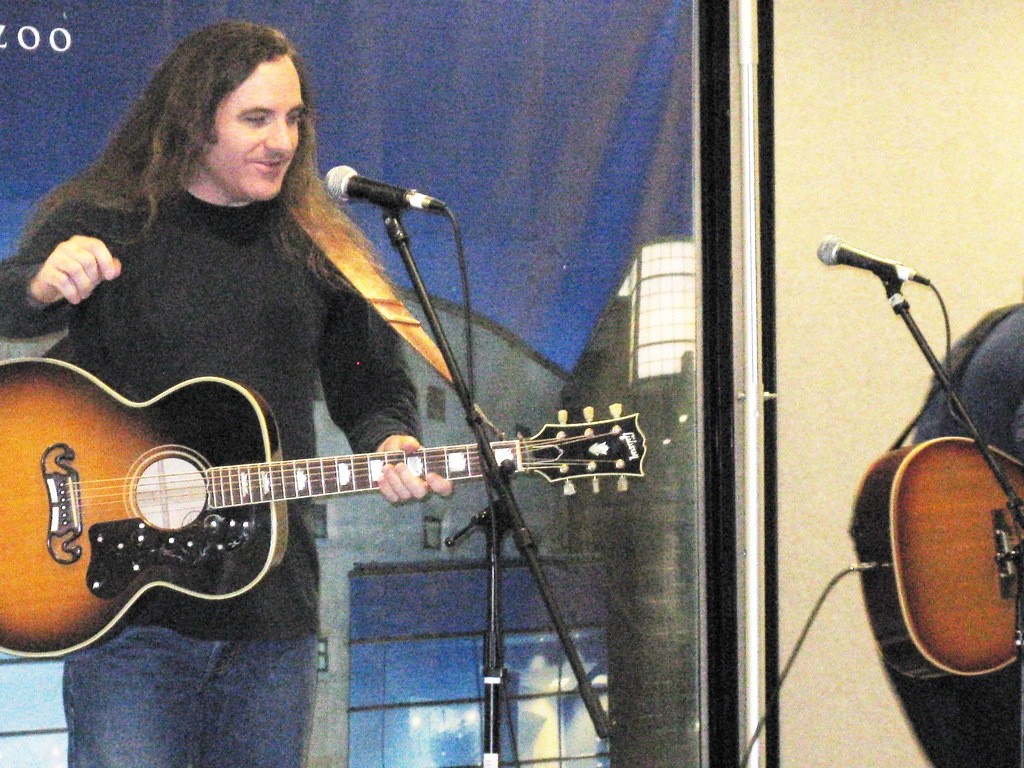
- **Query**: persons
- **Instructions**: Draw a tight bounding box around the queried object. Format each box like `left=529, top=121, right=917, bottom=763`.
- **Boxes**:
left=911, top=302, right=1024, bottom=768
left=0, top=21, right=462, bottom=768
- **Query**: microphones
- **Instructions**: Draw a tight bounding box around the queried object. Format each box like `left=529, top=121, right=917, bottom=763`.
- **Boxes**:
left=324, top=164, right=448, bottom=214
left=817, top=235, right=931, bottom=286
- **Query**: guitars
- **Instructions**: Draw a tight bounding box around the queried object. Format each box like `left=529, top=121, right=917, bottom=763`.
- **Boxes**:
left=1, top=354, right=649, bottom=661
left=850, top=434, right=1024, bottom=680
